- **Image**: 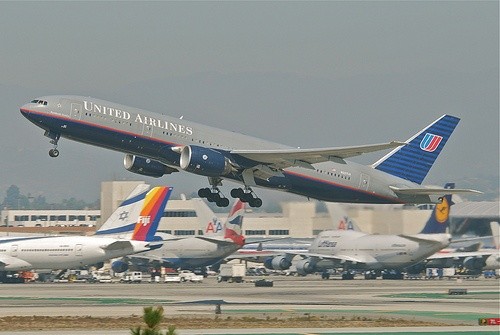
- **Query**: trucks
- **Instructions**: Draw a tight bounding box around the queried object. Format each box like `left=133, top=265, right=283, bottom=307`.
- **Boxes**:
left=216, top=263, right=246, bottom=283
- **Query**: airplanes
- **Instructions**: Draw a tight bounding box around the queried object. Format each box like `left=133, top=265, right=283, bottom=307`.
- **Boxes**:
left=225, top=181, right=500, bottom=281
left=112, top=196, right=247, bottom=270
left=20, top=92, right=484, bottom=212
left=423, top=220, right=500, bottom=276
left=0, top=181, right=175, bottom=284
